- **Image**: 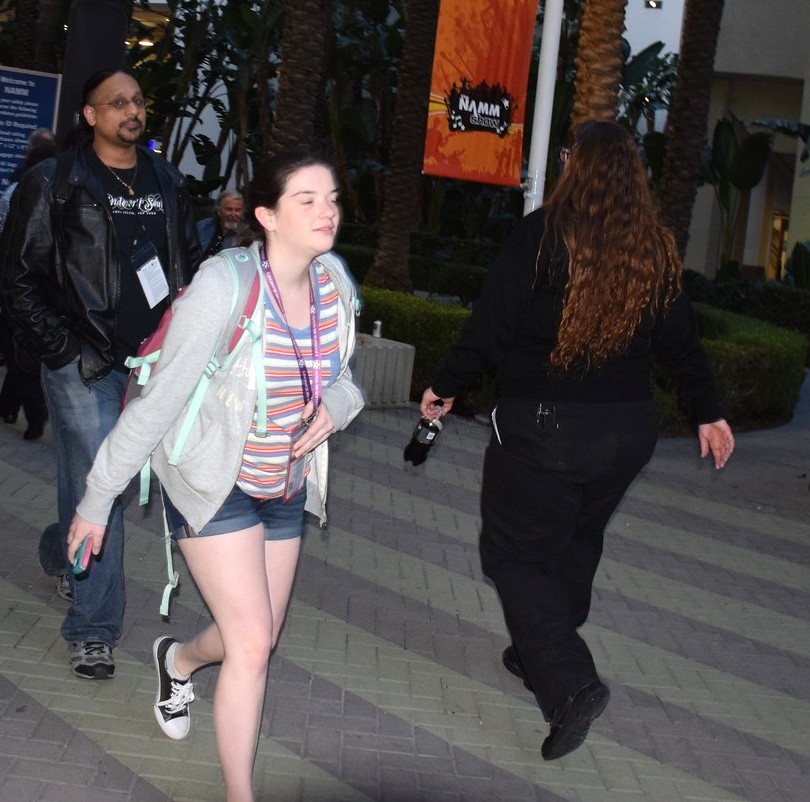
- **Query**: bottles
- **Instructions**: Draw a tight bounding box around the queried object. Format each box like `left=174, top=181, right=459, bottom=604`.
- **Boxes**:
left=403, top=401, right=444, bottom=465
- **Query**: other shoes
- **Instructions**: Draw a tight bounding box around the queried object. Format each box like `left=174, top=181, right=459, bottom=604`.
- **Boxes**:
left=503, top=644, right=534, bottom=693
left=0, top=397, right=17, bottom=423
left=24, top=404, right=49, bottom=441
left=541, top=681, right=611, bottom=760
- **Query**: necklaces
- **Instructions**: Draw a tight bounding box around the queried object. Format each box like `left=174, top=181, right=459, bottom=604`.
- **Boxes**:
left=96, top=153, right=138, bottom=196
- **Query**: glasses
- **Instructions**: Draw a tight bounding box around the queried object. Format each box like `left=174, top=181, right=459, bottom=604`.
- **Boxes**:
left=86, top=94, right=148, bottom=109
left=558, top=146, right=575, bottom=163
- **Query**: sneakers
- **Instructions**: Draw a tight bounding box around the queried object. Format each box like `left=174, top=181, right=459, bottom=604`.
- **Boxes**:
left=153, top=635, right=196, bottom=740
left=67, top=637, right=116, bottom=679
left=53, top=573, right=73, bottom=601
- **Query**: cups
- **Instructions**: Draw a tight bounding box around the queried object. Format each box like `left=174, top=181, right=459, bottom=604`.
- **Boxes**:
left=373, top=321, right=383, bottom=337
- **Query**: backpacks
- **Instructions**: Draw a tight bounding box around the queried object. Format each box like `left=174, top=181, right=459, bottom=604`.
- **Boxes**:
left=133, top=244, right=266, bottom=410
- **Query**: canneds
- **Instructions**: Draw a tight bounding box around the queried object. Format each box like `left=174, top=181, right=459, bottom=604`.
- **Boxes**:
left=373, top=321, right=383, bottom=339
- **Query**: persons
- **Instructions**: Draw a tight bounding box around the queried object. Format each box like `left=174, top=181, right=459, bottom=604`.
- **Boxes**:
left=420, top=119, right=735, bottom=760
left=0, top=127, right=58, bottom=439
left=66, top=158, right=366, bottom=802
left=196, top=191, right=253, bottom=259
left=0, top=65, right=205, bottom=679
